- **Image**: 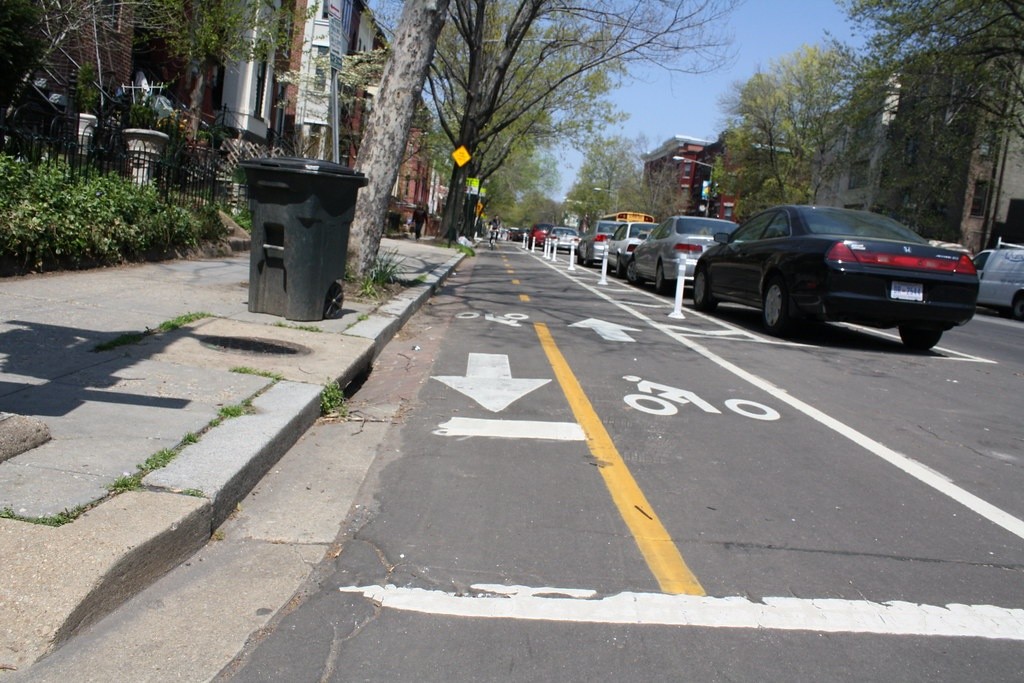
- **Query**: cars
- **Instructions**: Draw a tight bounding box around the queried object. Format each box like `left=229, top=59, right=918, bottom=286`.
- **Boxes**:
left=577, top=219, right=661, bottom=278
left=625, top=216, right=751, bottom=296
left=694, top=204, right=981, bottom=352
left=507, top=222, right=582, bottom=254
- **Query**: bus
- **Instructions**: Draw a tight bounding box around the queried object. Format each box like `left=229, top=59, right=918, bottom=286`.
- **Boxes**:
left=599, top=211, right=654, bottom=231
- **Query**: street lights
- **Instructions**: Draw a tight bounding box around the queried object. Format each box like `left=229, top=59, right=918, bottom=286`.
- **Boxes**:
left=593, top=187, right=618, bottom=213
left=673, top=156, right=714, bottom=218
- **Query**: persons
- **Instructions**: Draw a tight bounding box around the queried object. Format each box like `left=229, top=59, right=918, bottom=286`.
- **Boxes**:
left=412, top=202, right=428, bottom=241
left=489, top=215, right=500, bottom=244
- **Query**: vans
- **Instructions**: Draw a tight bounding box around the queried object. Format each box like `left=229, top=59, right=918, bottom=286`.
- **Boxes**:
left=970, top=239, right=1024, bottom=321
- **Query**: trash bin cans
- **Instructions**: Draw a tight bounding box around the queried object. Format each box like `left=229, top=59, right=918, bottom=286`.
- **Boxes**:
left=388, top=211, right=402, bottom=232
left=236, top=157, right=369, bottom=321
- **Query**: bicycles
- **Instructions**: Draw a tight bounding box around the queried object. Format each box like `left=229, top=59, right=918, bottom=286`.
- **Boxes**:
left=489, top=230, right=499, bottom=250
